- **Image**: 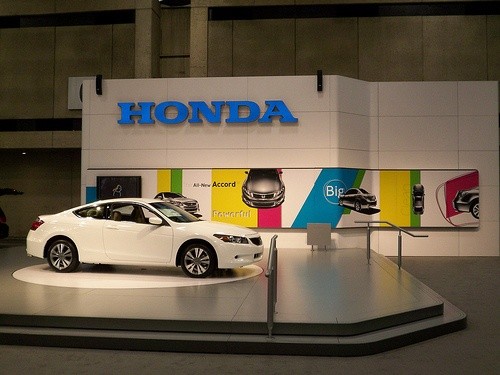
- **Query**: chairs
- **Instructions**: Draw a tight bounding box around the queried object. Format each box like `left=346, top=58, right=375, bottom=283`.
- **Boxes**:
left=110, top=211, right=122, bottom=221
left=130, top=207, right=145, bottom=223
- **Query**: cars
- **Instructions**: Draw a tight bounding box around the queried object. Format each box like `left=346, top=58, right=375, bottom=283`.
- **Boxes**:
left=339, top=186, right=379, bottom=210
left=412, top=184, right=425, bottom=215
left=154, top=192, right=199, bottom=213
left=451, top=185, right=479, bottom=220
left=240, top=169, right=287, bottom=209
left=23, top=195, right=265, bottom=278
